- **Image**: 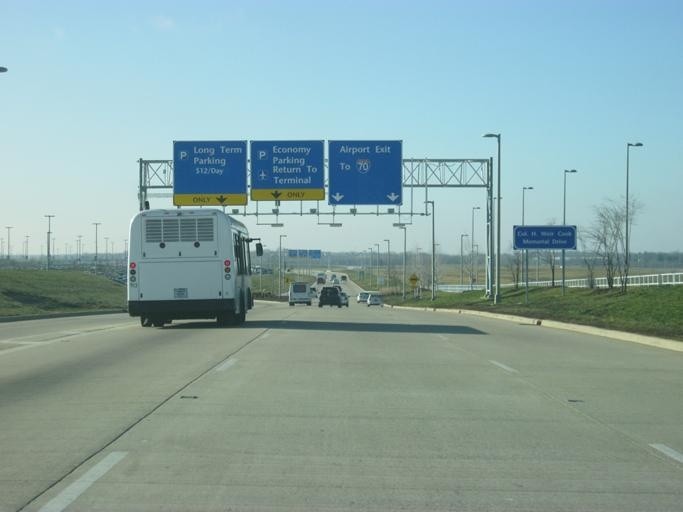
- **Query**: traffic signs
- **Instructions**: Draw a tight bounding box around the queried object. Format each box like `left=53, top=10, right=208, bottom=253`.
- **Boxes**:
left=512, top=224, right=577, bottom=251
left=171, top=141, right=247, bottom=206
left=249, top=140, right=325, bottom=201
left=327, top=140, right=403, bottom=206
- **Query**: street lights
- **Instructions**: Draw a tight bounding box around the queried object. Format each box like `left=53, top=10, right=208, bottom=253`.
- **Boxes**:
left=460, top=234, right=469, bottom=285
left=259, top=245, right=267, bottom=294
left=278, top=234, right=287, bottom=299
left=625, top=143, right=643, bottom=268
left=481, top=134, right=502, bottom=305
left=357, top=201, right=440, bottom=302
left=561, top=168, right=578, bottom=296
left=0, top=215, right=128, bottom=275
left=472, top=244, right=478, bottom=286
left=470, top=206, right=480, bottom=290
left=491, top=197, right=503, bottom=284
left=520, top=186, right=534, bottom=287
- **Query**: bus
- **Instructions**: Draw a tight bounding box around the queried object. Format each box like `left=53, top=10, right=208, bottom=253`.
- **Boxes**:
left=123, top=209, right=262, bottom=328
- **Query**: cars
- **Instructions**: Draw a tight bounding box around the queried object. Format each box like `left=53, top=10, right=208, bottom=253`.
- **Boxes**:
left=289, top=270, right=382, bottom=308
